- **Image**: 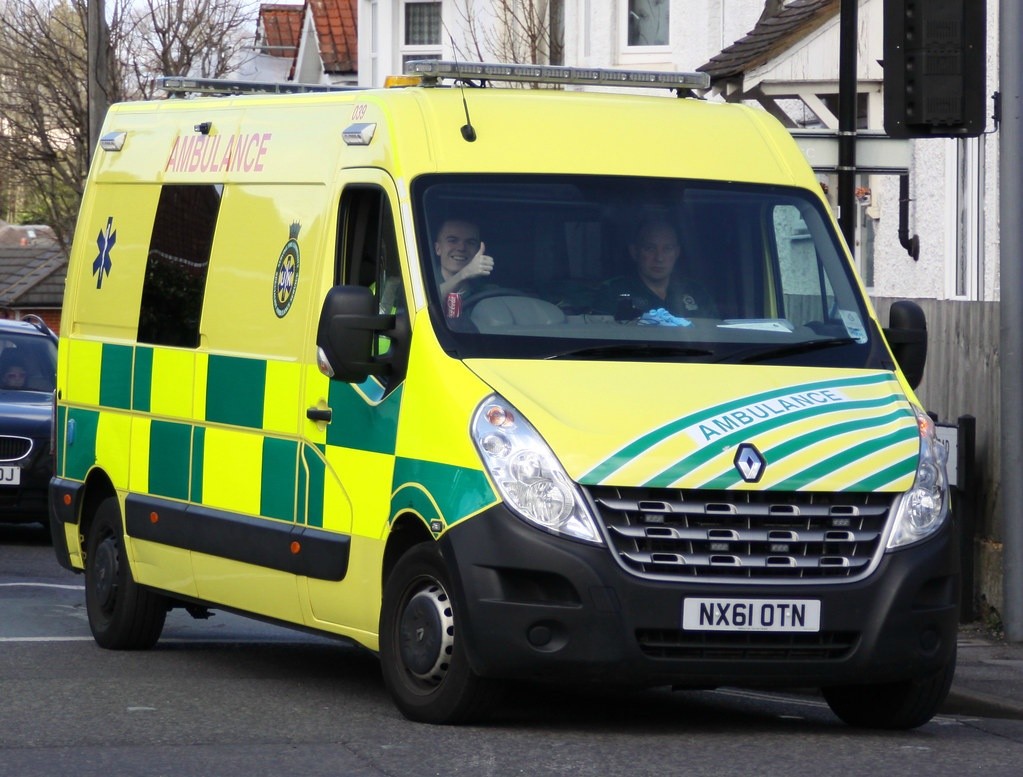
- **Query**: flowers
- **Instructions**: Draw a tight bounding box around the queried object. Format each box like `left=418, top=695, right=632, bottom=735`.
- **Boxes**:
left=857, top=187, right=871, bottom=199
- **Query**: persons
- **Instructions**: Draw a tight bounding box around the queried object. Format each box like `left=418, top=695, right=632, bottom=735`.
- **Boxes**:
left=1, top=347, right=29, bottom=390
left=588, top=218, right=723, bottom=321
left=395, top=215, right=505, bottom=326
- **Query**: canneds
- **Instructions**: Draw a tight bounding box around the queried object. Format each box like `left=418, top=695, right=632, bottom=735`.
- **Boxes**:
left=446, top=293, right=462, bottom=319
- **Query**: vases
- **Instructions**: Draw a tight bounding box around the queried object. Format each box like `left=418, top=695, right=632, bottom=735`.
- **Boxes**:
left=858, top=194, right=871, bottom=206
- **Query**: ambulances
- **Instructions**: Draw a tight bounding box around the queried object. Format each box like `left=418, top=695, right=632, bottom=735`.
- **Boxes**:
left=44, top=57, right=960, bottom=734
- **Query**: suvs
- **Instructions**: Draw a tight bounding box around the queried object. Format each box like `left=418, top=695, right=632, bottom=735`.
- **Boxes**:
left=0, top=314, right=59, bottom=547
